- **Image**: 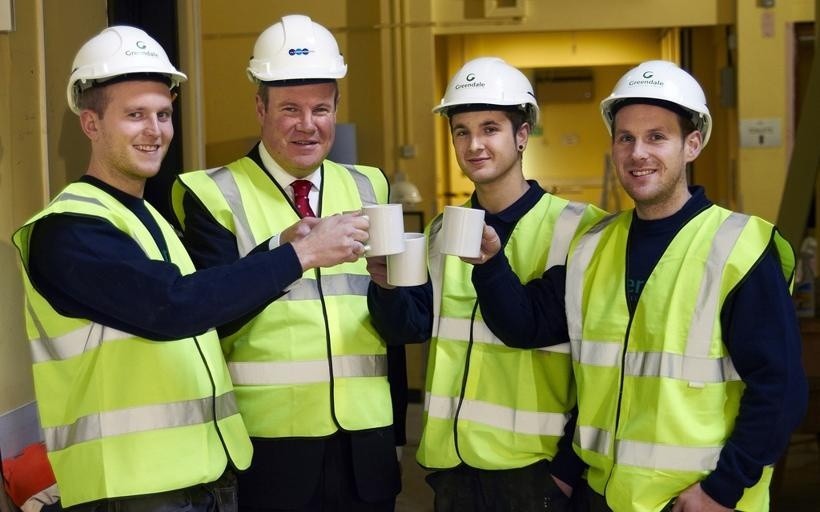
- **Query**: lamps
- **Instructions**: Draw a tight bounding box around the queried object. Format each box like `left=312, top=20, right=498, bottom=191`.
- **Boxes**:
left=386, top=170, right=422, bottom=205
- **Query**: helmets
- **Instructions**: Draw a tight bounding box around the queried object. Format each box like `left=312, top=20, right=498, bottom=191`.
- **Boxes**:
left=600, top=60, right=712, bottom=150
left=246, top=15, right=347, bottom=85
left=67, top=25, right=188, bottom=117
left=432, top=57, right=539, bottom=127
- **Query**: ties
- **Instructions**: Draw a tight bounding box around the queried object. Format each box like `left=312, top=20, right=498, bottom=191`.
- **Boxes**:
left=290, top=179, right=316, bottom=221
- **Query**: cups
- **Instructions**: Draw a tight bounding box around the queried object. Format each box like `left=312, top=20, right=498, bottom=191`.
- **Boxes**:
left=387, top=232, right=429, bottom=287
left=439, top=205, right=485, bottom=259
left=353, top=203, right=405, bottom=258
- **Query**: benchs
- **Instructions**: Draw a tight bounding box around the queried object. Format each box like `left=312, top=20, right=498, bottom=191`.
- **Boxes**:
left=1, top=400, right=113, bottom=512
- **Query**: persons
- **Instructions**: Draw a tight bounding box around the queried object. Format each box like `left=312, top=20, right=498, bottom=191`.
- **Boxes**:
left=10, top=25, right=370, bottom=510
left=164, top=1, right=432, bottom=512
left=365, top=55, right=594, bottom=510
left=451, top=58, right=797, bottom=512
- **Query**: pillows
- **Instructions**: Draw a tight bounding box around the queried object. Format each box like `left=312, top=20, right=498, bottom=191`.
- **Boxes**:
left=1, top=442, right=61, bottom=512
